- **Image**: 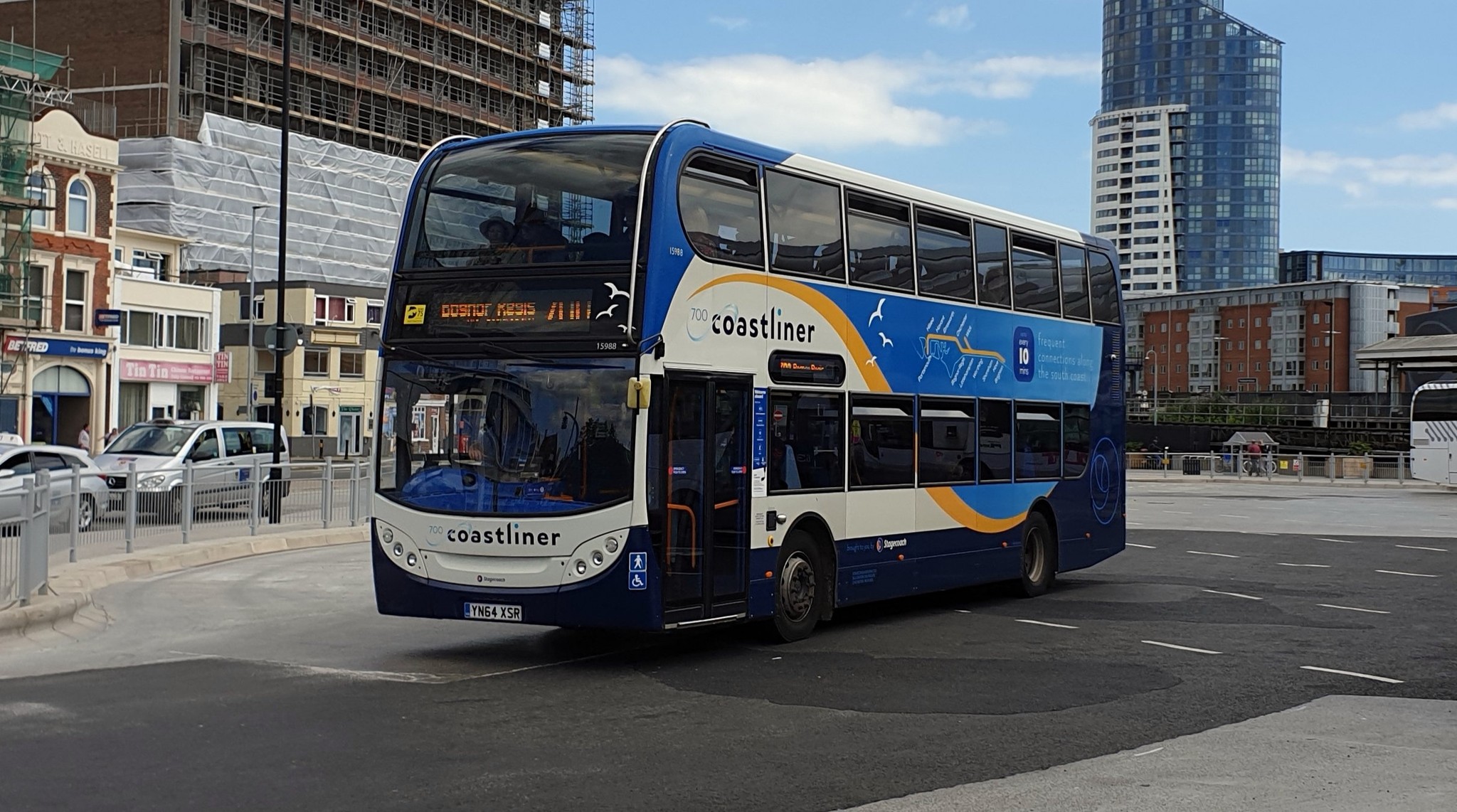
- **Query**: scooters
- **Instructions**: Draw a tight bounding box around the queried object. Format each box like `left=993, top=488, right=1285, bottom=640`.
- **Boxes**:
left=1141, top=447, right=1170, bottom=470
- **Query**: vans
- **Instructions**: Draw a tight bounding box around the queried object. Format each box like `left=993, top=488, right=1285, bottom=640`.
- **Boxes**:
left=94, top=418, right=293, bottom=522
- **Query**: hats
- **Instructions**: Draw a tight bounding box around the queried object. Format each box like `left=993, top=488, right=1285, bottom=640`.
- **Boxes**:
left=479, top=216, right=514, bottom=239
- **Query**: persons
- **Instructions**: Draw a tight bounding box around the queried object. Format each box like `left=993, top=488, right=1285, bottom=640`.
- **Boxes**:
left=444, top=205, right=643, bottom=303
left=684, top=203, right=717, bottom=258
left=1257, top=440, right=1267, bottom=476
left=1245, top=440, right=1261, bottom=477
left=545, top=403, right=633, bottom=504
left=1222, top=448, right=1231, bottom=468
left=734, top=215, right=759, bottom=242
left=1149, top=440, right=1163, bottom=470
left=78, top=423, right=90, bottom=452
left=929, top=454, right=995, bottom=482
left=97, top=428, right=119, bottom=445
left=468, top=403, right=530, bottom=469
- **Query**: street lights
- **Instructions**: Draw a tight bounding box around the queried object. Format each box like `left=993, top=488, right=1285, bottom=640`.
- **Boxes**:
left=1145, top=350, right=1157, bottom=426
left=1322, top=300, right=1333, bottom=450
left=247, top=205, right=272, bottom=419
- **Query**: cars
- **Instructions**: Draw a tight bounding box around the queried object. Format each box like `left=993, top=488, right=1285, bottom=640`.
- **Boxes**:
left=0, top=432, right=110, bottom=532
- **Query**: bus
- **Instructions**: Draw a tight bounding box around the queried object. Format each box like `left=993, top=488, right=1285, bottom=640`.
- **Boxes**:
left=368, top=119, right=1126, bottom=642
left=1410, top=379, right=1457, bottom=486
left=823, top=409, right=1060, bottom=477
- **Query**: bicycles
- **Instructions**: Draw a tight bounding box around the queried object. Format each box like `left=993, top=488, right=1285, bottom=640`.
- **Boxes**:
left=1215, top=458, right=1237, bottom=473
left=1244, top=458, right=1278, bottom=473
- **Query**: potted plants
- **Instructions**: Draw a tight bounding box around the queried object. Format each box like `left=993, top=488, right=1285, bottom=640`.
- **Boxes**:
left=1324, top=440, right=1373, bottom=479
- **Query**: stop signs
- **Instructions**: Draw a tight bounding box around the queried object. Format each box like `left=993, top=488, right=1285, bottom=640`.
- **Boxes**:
left=774, top=410, right=783, bottom=422
